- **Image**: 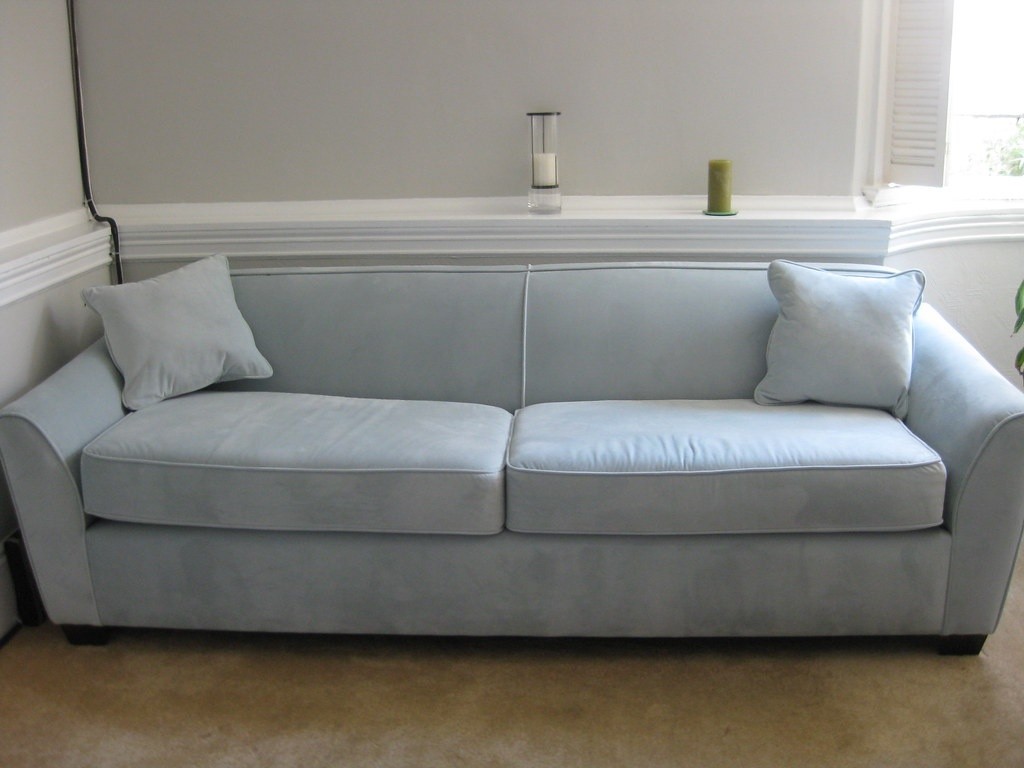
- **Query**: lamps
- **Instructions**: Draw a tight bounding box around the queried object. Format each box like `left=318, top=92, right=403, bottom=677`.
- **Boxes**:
left=527, top=112, right=562, bottom=214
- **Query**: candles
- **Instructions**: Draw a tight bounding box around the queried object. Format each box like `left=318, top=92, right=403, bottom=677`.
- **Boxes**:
left=707, top=160, right=733, bottom=212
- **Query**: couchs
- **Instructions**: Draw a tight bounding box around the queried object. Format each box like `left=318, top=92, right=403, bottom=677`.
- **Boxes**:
left=1, top=263, right=1024, bottom=652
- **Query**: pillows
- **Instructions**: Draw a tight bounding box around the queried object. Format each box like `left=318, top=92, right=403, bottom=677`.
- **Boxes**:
left=81, top=253, right=274, bottom=409
left=754, top=260, right=925, bottom=423
